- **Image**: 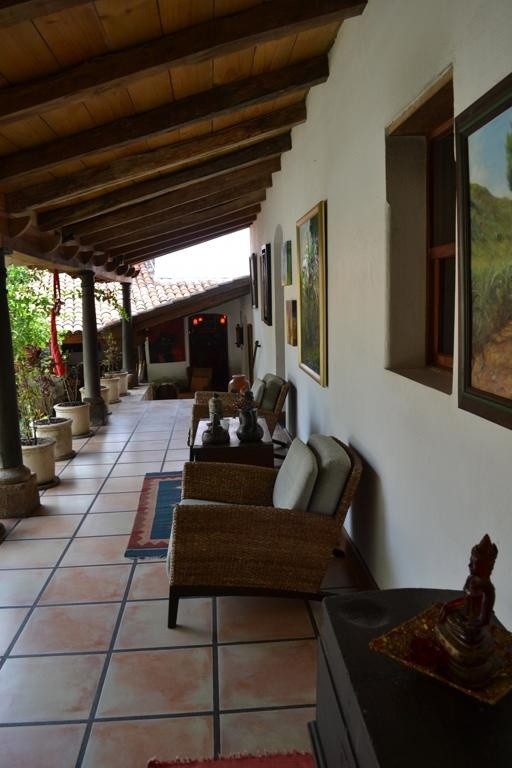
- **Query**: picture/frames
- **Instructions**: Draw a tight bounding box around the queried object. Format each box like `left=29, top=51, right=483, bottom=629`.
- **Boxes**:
left=249, top=200, right=328, bottom=388
left=455, top=72, right=512, bottom=430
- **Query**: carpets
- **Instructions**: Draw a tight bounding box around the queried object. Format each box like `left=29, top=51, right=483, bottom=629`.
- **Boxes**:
left=147, top=751, right=317, bottom=768
left=124, top=471, right=186, bottom=560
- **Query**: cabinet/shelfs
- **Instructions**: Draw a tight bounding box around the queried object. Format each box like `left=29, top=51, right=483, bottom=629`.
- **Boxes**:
left=307, top=588, right=512, bottom=768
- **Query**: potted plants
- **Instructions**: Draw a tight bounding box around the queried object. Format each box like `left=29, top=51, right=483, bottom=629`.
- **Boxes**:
left=14, top=329, right=131, bottom=490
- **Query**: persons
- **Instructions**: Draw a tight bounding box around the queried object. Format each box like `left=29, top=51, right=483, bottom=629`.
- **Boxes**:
left=433, top=532, right=499, bottom=668
